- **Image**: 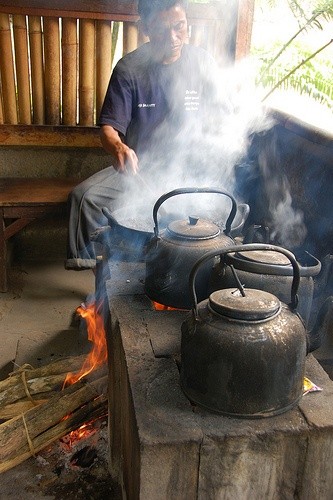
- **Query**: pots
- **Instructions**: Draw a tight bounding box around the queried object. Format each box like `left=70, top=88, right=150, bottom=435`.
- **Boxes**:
left=99, top=194, right=250, bottom=241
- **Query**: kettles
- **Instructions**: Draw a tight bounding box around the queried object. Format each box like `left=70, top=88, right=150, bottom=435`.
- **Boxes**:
left=145, top=187, right=240, bottom=311
left=177, top=244, right=308, bottom=420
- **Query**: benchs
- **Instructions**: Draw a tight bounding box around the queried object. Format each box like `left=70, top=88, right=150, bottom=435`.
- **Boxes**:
left=0, top=179, right=91, bottom=295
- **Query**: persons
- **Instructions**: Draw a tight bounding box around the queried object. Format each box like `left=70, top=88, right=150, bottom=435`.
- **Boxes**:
left=66, top=0, right=247, bottom=280
